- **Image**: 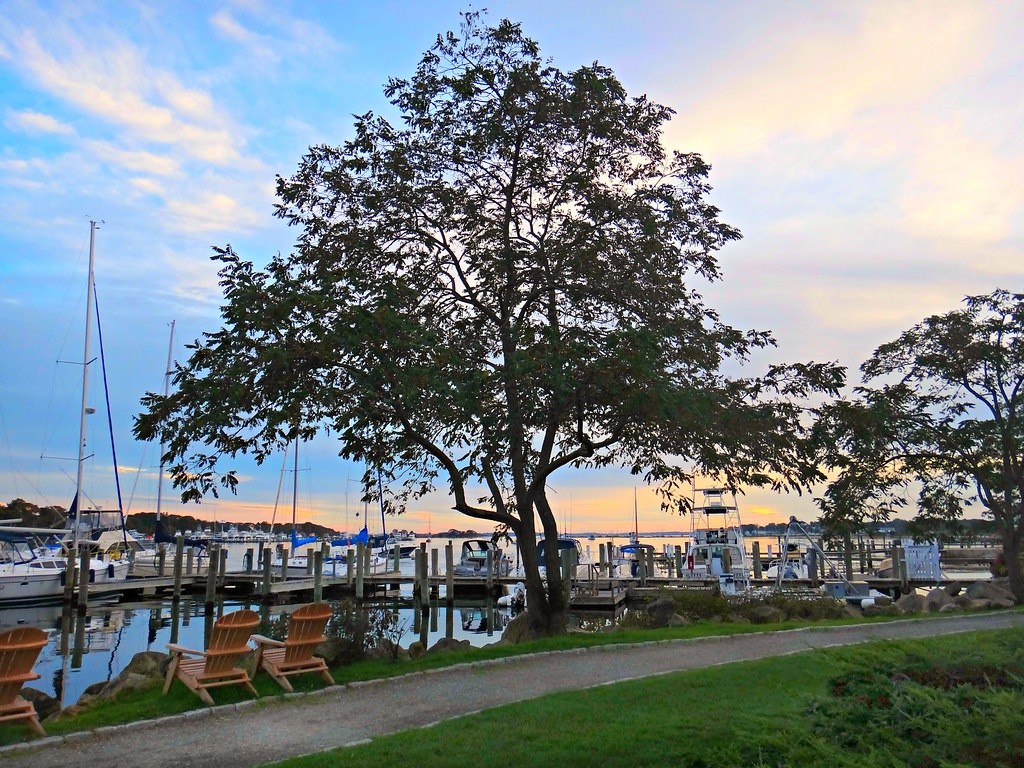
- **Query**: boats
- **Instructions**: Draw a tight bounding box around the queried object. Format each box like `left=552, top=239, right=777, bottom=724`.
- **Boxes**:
left=768, top=558, right=803, bottom=578
left=680, top=484, right=751, bottom=577
left=453, top=539, right=513, bottom=577
left=519, top=538, right=596, bottom=578
left=878, top=559, right=895, bottom=578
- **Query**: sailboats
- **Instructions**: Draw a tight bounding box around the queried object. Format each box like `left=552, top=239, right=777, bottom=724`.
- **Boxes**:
left=1, top=216, right=420, bottom=605
left=605, top=486, right=662, bottom=576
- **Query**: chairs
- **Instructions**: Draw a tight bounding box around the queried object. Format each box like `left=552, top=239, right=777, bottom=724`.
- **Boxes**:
left=0, top=627, right=48, bottom=738
left=251, top=602, right=335, bottom=693
left=161, top=610, right=260, bottom=707
